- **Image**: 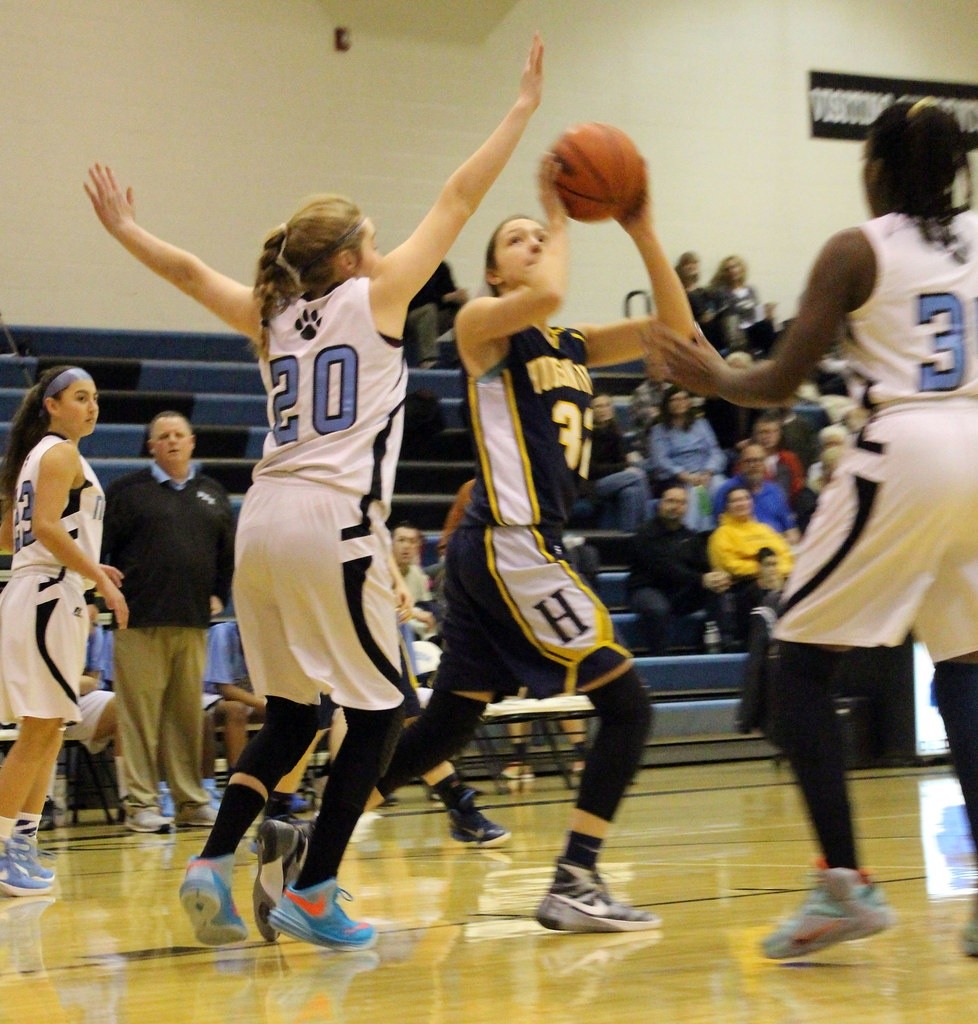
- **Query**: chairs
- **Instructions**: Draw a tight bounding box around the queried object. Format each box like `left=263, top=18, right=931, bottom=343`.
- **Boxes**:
left=213, top=725, right=308, bottom=803
left=478, top=699, right=572, bottom=793
left=536, top=696, right=639, bottom=786
left=0, top=726, right=116, bottom=828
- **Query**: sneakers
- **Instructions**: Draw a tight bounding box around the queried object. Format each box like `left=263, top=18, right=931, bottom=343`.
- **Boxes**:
left=180, top=854, right=246, bottom=946
left=758, top=859, right=896, bottom=959
left=536, top=857, right=663, bottom=932
left=125, top=810, right=170, bottom=833
left=253, top=820, right=308, bottom=941
left=0, top=835, right=51, bottom=897
left=205, top=788, right=225, bottom=812
left=175, top=805, right=219, bottom=826
left=160, top=788, right=176, bottom=817
left=267, top=878, right=378, bottom=951
left=291, top=796, right=311, bottom=813
left=450, top=794, right=512, bottom=846
left=11, top=834, right=55, bottom=882
left=958, top=896, right=978, bottom=957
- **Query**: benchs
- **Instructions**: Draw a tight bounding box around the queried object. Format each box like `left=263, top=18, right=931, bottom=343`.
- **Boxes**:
left=0, top=324, right=884, bottom=812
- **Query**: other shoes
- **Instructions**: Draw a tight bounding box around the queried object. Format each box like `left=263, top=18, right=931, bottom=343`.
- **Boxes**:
left=38, top=815, right=53, bottom=829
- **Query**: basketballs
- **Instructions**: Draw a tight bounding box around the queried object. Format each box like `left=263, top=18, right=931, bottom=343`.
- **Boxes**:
left=550, top=122, right=641, bottom=225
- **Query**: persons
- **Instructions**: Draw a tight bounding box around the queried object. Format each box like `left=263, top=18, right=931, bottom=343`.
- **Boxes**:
left=42, top=594, right=510, bottom=848
left=405, top=259, right=472, bottom=371
left=84, top=411, right=237, bottom=834
left=389, top=471, right=478, bottom=682
left=632, top=346, right=876, bottom=736
left=642, top=98, right=978, bottom=961
left=1, top=365, right=129, bottom=897
left=585, top=394, right=655, bottom=537
left=85, top=34, right=546, bottom=951
left=668, top=250, right=798, bottom=362
left=253, top=153, right=694, bottom=947
left=493, top=692, right=586, bottom=778
